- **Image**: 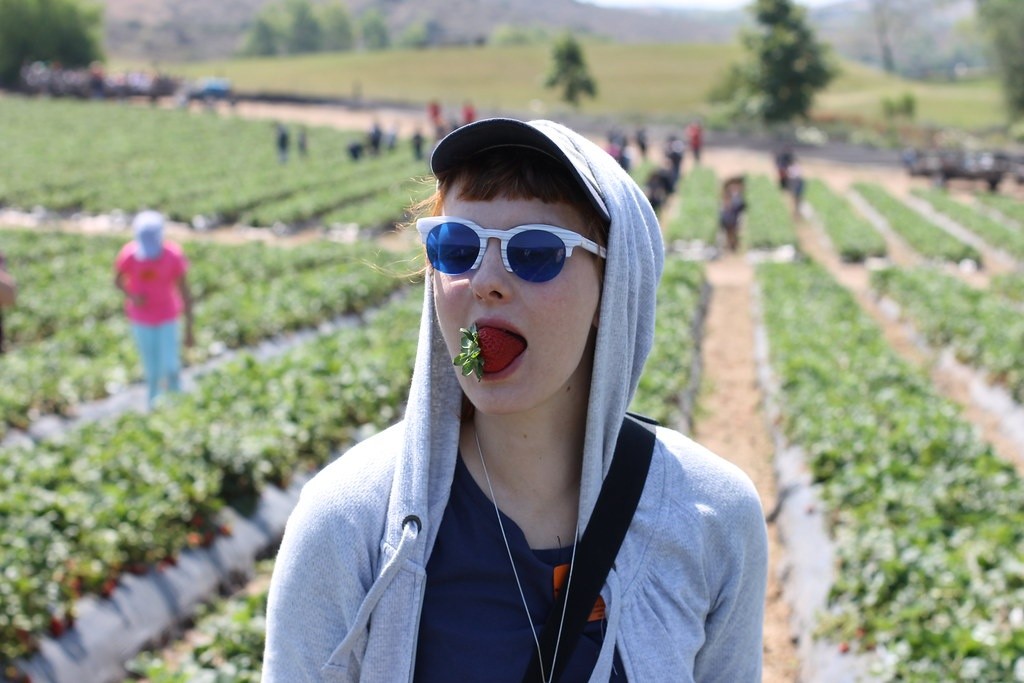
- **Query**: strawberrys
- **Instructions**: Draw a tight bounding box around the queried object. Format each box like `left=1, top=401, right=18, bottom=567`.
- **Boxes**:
left=0, top=514, right=231, bottom=640
left=836, top=625, right=876, bottom=655
left=451, top=322, right=525, bottom=382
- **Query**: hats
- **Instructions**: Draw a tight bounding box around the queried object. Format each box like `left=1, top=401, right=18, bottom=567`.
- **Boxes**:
left=132, top=211, right=165, bottom=259
left=431, top=117, right=608, bottom=215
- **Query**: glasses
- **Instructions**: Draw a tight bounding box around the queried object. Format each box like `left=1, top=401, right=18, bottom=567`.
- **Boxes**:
left=416, top=215, right=607, bottom=283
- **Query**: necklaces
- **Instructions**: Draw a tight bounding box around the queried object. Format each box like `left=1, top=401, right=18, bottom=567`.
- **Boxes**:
left=473, top=434, right=579, bottom=683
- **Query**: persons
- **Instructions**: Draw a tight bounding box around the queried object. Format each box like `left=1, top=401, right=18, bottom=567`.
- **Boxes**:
left=348, top=100, right=475, bottom=164
left=260, top=118, right=770, bottom=683
left=112, top=211, right=196, bottom=410
left=274, top=123, right=288, bottom=167
left=296, top=124, right=306, bottom=156
left=606, top=121, right=804, bottom=252
